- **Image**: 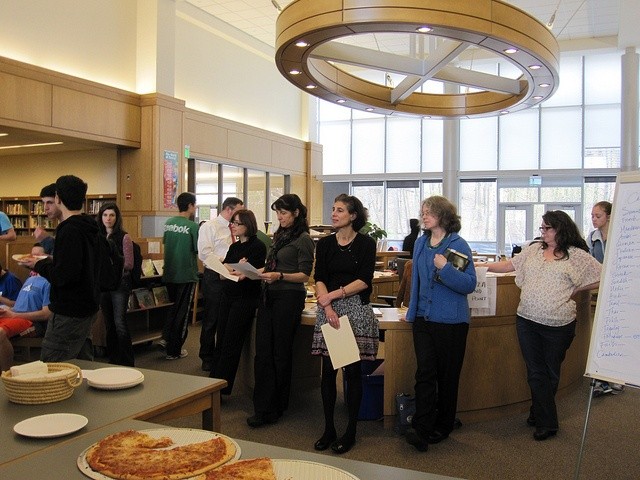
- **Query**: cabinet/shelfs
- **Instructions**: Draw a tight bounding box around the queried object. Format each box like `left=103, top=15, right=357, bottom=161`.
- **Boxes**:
left=1, top=193, right=117, bottom=237
left=92, top=238, right=170, bottom=355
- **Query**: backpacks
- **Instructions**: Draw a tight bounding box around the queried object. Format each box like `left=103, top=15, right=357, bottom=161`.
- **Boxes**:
left=127, top=242, right=144, bottom=291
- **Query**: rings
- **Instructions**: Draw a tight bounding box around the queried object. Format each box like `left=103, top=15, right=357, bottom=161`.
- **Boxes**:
left=265, top=280, right=266, bottom=282
left=330, top=318, right=332, bottom=321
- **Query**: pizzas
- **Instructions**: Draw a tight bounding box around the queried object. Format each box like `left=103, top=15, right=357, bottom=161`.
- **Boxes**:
left=190, top=456, right=278, bottom=480
left=18, top=253, right=36, bottom=260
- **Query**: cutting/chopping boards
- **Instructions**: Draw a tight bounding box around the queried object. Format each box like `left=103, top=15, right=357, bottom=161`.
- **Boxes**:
left=187, top=458, right=360, bottom=480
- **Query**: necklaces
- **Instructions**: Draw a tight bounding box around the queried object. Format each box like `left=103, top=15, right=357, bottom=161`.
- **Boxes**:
left=337, top=232, right=357, bottom=251
left=428, top=241, right=441, bottom=250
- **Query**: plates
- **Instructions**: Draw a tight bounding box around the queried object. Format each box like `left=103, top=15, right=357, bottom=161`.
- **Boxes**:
left=13, top=413, right=89, bottom=438
left=86, top=367, right=145, bottom=390
left=11, top=253, right=50, bottom=268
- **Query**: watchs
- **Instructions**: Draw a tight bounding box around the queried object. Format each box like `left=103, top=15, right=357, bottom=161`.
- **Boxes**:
left=340, top=286, right=346, bottom=299
left=279, top=272, right=284, bottom=280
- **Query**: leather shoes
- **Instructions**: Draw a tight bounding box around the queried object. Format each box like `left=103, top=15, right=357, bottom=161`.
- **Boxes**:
left=430, top=428, right=452, bottom=445
left=534, top=426, right=560, bottom=441
left=247, top=411, right=278, bottom=428
left=313, top=429, right=337, bottom=450
left=400, top=425, right=429, bottom=453
left=332, top=435, right=356, bottom=454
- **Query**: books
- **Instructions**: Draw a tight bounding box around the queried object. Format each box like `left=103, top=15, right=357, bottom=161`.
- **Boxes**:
left=10, top=217, right=27, bottom=228
left=435, top=248, right=470, bottom=287
left=141, top=258, right=165, bottom=278
left=87, top=199, right=107, bottom=214
left=6, top=201, right=27, bottom=215
left=31, top=216, right=60, bottom=228
left=127, top=286, right=171, bottom=311
left=30, top=200, right=47, bottom=215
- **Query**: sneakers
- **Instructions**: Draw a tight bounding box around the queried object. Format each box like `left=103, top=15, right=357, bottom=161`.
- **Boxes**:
left=151, top=339, right=167, bottom=350
left=166, top=349, right=188, bottom=360
left=591, top=381, right=612, bottom=396
left=612, top=383, right=626, bottom=395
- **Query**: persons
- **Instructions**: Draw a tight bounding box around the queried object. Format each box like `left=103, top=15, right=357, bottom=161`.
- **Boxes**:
left=406, top=196, right=476, bottom=452
left=246, top=194, right=315, bottom=428
left=197, top=198, right=244, bottom=371
left=0, top=242, right=54, bottom=377
left=0, top=264, right=23, bottom=307
left=314, top=193, right=379, bottom=453
left=20, top=175, right=105, bottom=363
left=474, top=210, right=602, bottom=441
left=97, top=202, right=134, bottom=367
left=33, top=183, right=63, bottom=255
left=208, top=209, right=267, bottom=403
left=0, top=211, right=17, bottom=241
left=585, top=201, right=612, bottom=264
left=152, top=192, right=200, bottom=360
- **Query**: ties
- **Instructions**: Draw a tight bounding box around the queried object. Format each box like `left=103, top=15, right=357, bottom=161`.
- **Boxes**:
left=228, top=223, right=236, bottom=244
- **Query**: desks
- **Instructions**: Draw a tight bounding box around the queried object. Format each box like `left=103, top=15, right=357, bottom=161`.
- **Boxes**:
left=1, top=418, right=464, bottom=480
left=1, top=358, right=229, bottom=465
left=301, top=251, right=592, bottom=431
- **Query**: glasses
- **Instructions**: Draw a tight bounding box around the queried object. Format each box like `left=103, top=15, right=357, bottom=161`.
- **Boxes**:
left=231, top=221, right=244, bottom=227
left=419, top=210, right=433, bottom=216
left=537, top=227, right=554, bottom=231
